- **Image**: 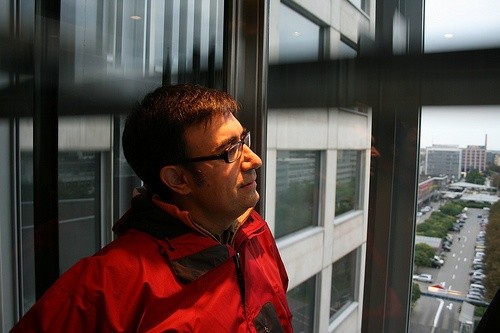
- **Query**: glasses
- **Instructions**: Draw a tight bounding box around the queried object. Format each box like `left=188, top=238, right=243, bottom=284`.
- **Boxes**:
left=173, top=131, right=252, bottom=165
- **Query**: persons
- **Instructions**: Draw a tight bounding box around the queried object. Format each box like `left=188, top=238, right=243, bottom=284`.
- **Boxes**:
left=8, top=84, right=296, bottom=333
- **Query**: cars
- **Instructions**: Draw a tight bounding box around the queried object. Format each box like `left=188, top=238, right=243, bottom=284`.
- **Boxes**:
left=444, top=212, right=468, bottom=257
left=466, top=207, right=490, bottom=303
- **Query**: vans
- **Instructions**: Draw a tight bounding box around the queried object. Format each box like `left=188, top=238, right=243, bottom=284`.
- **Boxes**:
left=427, top=257, right=444, bottom=270
left=413, top=274, right=433, bottom=283
